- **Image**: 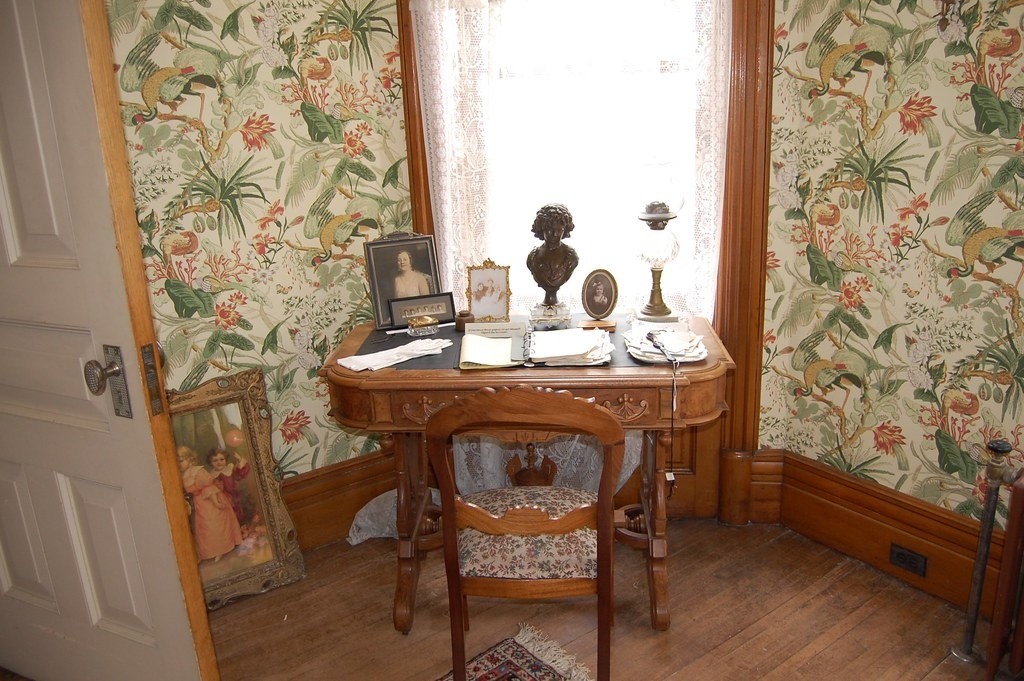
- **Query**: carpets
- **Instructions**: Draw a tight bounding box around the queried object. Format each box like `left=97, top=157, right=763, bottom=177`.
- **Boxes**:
left=435, top=623, right=596, bottom=681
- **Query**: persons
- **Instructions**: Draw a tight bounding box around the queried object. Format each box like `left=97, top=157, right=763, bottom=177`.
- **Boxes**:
left=526, top=204, right=578, bottom=292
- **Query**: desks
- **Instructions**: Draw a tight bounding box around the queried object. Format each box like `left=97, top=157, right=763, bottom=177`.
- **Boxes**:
left=318, top=317, right=737, bottom=635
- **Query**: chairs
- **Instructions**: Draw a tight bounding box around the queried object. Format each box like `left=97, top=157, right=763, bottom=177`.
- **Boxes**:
left=422, top=383, right=627, bottom=681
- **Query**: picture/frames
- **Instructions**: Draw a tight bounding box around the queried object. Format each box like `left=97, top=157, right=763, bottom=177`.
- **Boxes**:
left=388, top=291, right=455, bottom=328
left=463, top=256, right=514, bottom=322
left=581, top=269, right=619, bottom=320
left=165, top=364, right=305, bottom=614
left=363, top=232, right=441, bottom=331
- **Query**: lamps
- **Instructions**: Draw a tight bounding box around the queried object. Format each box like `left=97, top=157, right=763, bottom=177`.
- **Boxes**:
left=636, top=202, right=679, bottom=316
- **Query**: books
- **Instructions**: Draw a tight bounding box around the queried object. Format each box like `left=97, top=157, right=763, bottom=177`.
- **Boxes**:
left=452, top=328, right=616, bottom=371
